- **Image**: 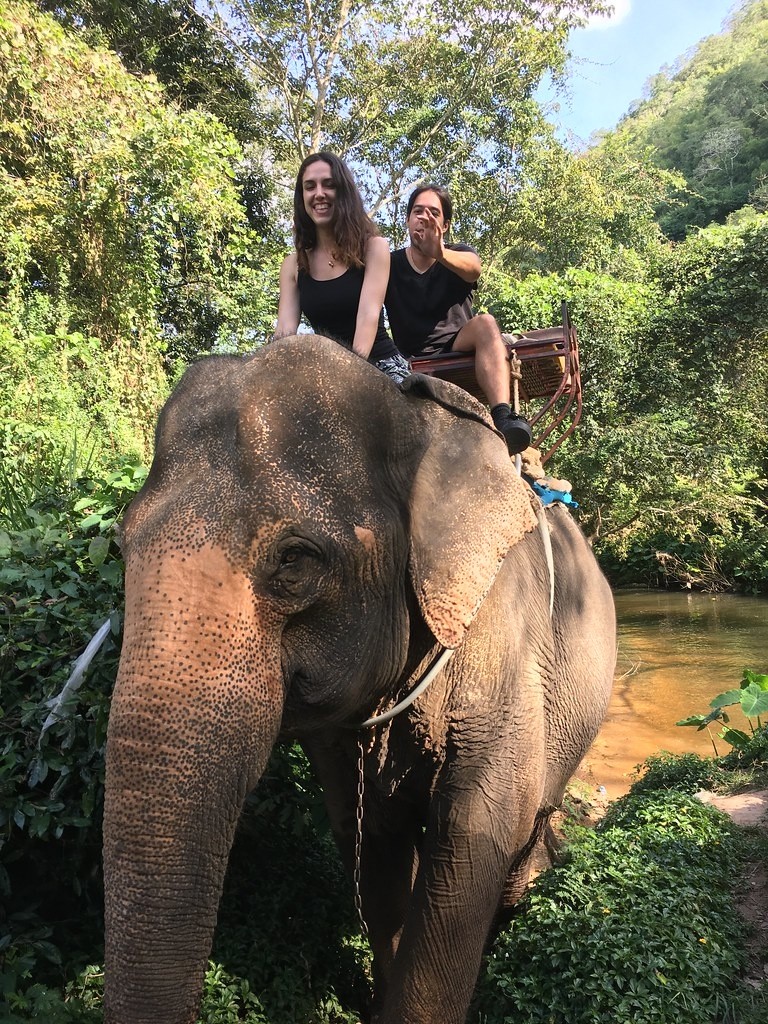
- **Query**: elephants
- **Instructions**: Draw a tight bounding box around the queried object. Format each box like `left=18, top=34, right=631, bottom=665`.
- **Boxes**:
left=103, top=335, right=617, bottom=1024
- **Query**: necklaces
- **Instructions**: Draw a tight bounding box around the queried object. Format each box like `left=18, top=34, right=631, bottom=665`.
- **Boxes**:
left=321, top=246, right=335, bottom=268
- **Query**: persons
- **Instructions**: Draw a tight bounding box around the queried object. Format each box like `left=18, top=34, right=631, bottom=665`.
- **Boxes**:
left=272, top=152, right=413, bottom=392
left=383, top=183, right=534, bottom=457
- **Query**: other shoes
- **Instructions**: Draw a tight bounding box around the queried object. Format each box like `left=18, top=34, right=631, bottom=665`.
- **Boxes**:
left=495, top=409, right=532, bottom=457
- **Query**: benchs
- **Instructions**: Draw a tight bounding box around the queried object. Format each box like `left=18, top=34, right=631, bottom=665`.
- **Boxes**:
left=404, top=300, right=584, bottom=464
